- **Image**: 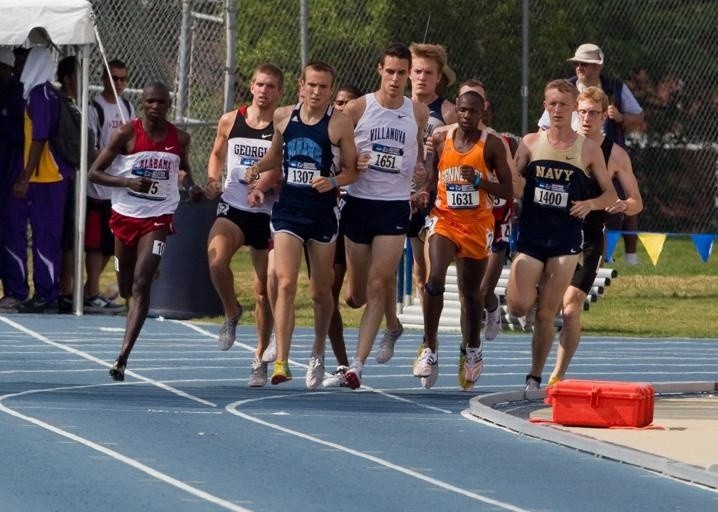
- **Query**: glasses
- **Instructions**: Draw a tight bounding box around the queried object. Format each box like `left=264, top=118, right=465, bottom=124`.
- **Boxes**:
left=576, top=110, right=605, bottom=117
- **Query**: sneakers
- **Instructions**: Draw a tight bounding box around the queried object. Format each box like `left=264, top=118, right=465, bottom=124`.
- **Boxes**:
left=109, top=359, right=126, bottom=381
left=0, top=292, right=126, bottom=313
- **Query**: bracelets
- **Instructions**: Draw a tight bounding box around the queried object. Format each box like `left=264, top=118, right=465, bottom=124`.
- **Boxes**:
left=253, top=166, right=260, bottom=179
left=207, top=179, right=215, bottom=184
left=329, top=177, right=338, bottom=187
left=625, top=201, right=630, bottom=211
left=418, top=192, right=428, bottom=196
left=474, top=176, right=481, bottom=188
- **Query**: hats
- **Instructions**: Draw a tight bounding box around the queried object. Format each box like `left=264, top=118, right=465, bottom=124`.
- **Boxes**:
left=566, top=43, right=604, bottom=64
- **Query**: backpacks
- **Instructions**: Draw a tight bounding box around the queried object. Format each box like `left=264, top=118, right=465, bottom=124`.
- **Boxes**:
left=28, top=81, right=94, bottom=165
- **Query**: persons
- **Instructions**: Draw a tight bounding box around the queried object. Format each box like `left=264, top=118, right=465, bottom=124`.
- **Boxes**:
left=1, top=43, right=135, bottom=312
left=411, top=79, right=511, bottom=381
left=204, top=64, right=289, bottom=388
left=418, top=77, right=523, bottom=389
left=370, top=41, right=457, bottom=366
left=427, top=60, right=462, bottom=126
left=243, top=58, right=360, bottom=392
left=467, top=99, right=525, bottom=341
left=87, top=82, right=205, bottom=382
left=549, top=86, right=646, bottom=385
left=539, top=43, right=648, bottom=265
left=303, top=85, right=369, bottom=386
left=339, top=45, right=430, bottom=387
left=511, top=80, right=619, bottom=399
